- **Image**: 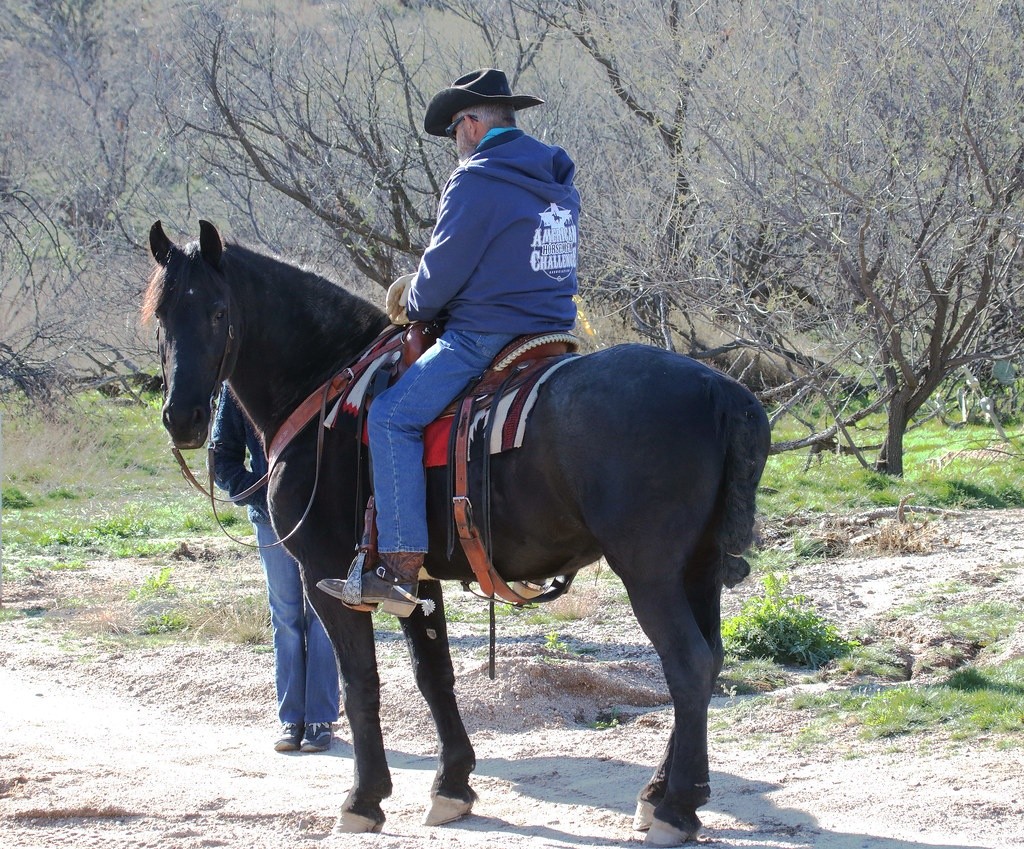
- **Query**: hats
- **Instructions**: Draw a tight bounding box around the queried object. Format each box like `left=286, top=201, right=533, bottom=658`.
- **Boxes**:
left=424, top=68, right=545, bottom=135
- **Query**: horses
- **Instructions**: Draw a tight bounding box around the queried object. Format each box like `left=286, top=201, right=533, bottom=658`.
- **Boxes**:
left=135, top=217, right=773, bottom=849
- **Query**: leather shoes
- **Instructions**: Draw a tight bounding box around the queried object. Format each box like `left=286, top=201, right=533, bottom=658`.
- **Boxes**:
left=316, top=567, right=420, bottom=616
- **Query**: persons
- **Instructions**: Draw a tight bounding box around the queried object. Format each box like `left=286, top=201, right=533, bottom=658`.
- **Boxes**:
left=314, top=68, right=581, bottom=617
left=207, top=381, right=340, bottom=753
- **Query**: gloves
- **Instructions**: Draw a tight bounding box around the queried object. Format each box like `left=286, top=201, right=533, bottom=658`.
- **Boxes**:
left=385, top=272, right=418, bottom=326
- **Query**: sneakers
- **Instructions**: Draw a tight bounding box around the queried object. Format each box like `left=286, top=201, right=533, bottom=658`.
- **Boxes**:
left=302, top=721, right=336, bottom=752
left=276, top=715, right=299, bottom=753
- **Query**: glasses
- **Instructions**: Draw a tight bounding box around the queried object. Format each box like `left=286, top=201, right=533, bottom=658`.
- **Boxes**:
left=444, top=112, right=481, bottom=138
